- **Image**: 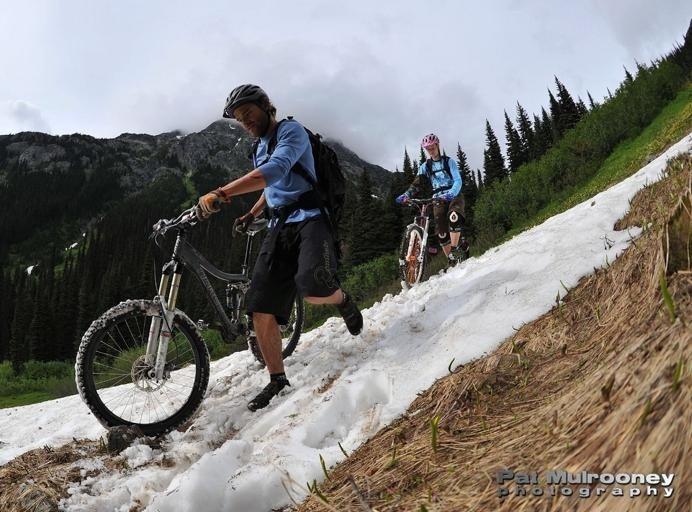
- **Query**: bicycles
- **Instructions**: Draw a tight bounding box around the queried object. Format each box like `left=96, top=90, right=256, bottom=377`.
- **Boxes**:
left=70, top=194, right=305, bottom=434
left=395, top=194, right=441, bottom=288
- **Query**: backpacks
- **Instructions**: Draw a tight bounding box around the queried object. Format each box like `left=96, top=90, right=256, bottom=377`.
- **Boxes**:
left=252, top=119, right=344, bottom=214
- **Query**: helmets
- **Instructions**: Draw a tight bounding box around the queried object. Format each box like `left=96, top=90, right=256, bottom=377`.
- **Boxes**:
left=222, top=84, right=269, bottom=119
left=421, top=134, right=440, bottom=148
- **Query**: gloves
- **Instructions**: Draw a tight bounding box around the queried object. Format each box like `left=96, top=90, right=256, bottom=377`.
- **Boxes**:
left=197, top=187, right=231, bottom=222
left=396, top=193, right=410, bottom=205
left=231, top=212, right=255, bottom=238
left=438, top=192, right=453, bottom=202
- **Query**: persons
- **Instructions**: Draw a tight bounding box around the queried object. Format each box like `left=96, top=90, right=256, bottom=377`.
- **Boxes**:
left=196, top=84, right=363, bottom=411
left=456, top=237, right=469, bottom=259
left=396, top=133, right=465, bottom=273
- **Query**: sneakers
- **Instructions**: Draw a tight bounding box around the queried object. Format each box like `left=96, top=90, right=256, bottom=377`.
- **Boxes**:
left=247, top=379, right=291, bottom=411
left=443, top=248, right=462, bottom=274
left=338, top=292, right=362, bottom=336
